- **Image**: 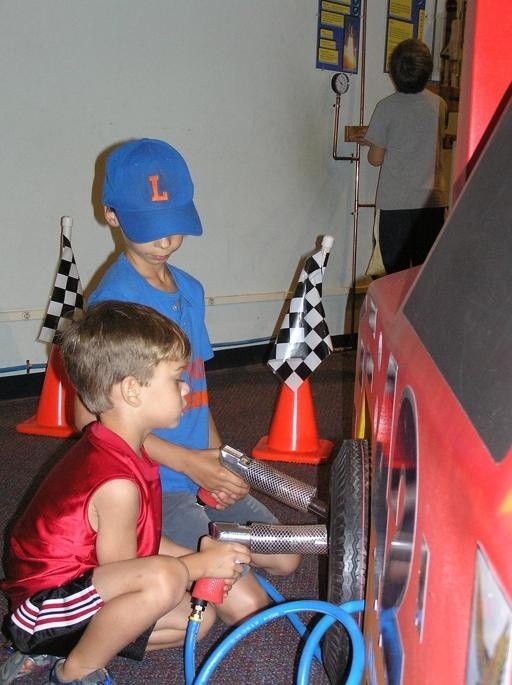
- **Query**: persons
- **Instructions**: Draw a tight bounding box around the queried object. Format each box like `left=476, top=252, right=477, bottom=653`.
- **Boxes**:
left=363, top=38, right=448, bottom=279
left=82, top=135, right=304, bottom=634
left=0, top=300, right=253, bottom=684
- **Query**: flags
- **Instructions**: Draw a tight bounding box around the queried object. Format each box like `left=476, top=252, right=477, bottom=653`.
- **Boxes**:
left=264, top=247, right=335, bottom=394
left=35, top=236, right=89, bottom=346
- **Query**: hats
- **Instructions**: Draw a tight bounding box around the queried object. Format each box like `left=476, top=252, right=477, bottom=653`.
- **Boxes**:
left=103, top=139, right=202, bottom=243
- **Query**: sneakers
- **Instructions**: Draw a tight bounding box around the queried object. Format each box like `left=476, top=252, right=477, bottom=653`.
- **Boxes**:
left=0, top=648, right=66, bottom=685
left=51, top=658, right=119, bottom=685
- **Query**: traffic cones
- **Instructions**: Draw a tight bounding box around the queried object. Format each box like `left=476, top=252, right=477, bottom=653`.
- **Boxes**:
left=249, top=362, right=333, bottom=463
left=14, top=344, right=80, bottom=439
